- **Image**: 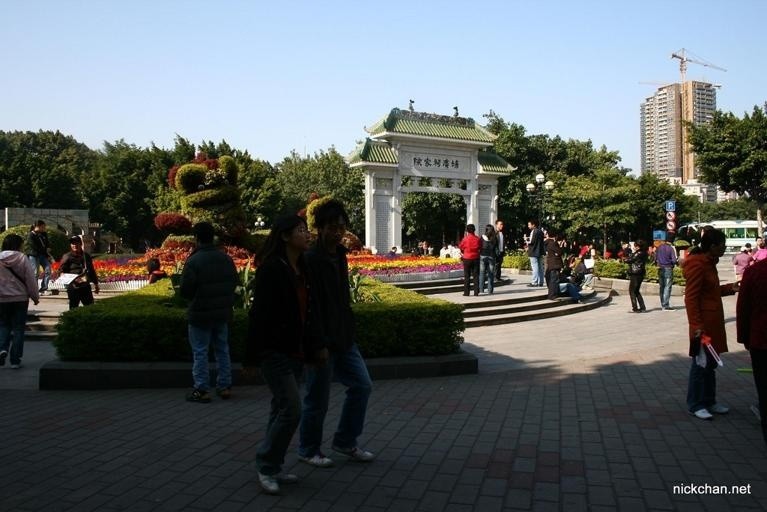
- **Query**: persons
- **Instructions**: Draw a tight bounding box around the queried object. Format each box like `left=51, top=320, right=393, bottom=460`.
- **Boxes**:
left=146, top=258, right=166, bottom=285
left=177, top=220, right=239, bottom=403
left=735, top=257, right=766, bottom=440
left=383, top=216, right=766, bottom=315
left=246, top=214, right=311, bottom=494
left=58, top=234, right=101, bottom=309
left=27, top=219, right=52, bottom=296
left=677, top=228, right=740, bottom=419
left=0, top=233, right=40, bottom=368
left=296, top=197, right=376, bottom=468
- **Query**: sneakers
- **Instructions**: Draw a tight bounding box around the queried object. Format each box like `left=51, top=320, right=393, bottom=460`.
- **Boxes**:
left=186, top=390, right=210, bottom=403
left=296, top=454, right=331, bottom=469
left=527, top=283, right=543, bottom=286
left=0, top=351, right=19, bottom=367
left=627, top=309, right=647, bottom=313
left=217, top=384, right=230, bottom=398
left=332, top=444, right=374, bottom=462
left=257, top=469, right=298, bottom=491
left=687, top=409, right=712, bottom=418
left=711, top=404, right=727, bottom=414
left=664, top=307, right=677, bottom=312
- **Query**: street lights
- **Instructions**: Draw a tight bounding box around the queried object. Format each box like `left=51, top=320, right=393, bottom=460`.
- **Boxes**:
left=525, top=172, right=556, bottom=233
left=254, top=216, right=266, bottom=232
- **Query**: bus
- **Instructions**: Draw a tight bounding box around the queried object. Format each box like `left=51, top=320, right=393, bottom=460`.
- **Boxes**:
left=676, top=219, right=767, bottom=252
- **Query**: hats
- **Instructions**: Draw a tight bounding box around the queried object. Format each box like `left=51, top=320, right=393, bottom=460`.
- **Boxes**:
left=70, top=235, right=82, bottom=244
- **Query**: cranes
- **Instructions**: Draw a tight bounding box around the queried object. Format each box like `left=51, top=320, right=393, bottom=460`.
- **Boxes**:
left=670, top=46, right=729, bottom=184
